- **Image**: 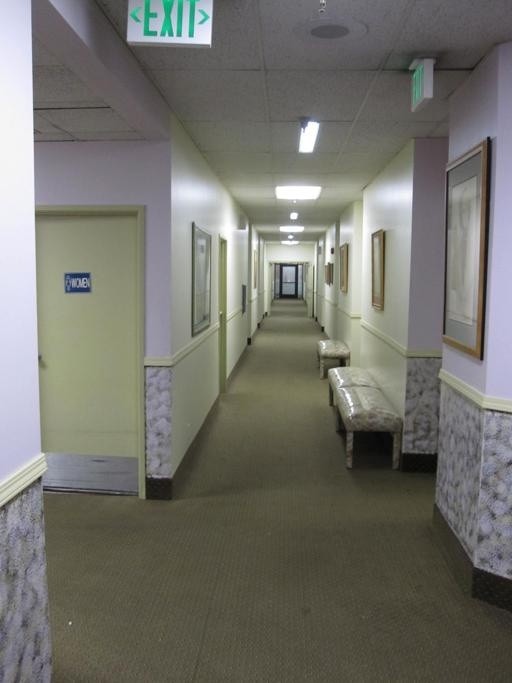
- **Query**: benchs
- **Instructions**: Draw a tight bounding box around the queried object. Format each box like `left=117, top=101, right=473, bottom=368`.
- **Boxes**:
left=327, top=366, right=405, bottom=471
left=316, top=339, right=351, bottom=379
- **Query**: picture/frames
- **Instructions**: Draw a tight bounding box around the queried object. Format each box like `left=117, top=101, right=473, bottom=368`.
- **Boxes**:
left=339, top=240, right=348, bottom=293
left=370, top=228, right=385, bottom=311
left=443, top=135, right=495, bottom=360
left=190, top=222, right=212, bottom=336
